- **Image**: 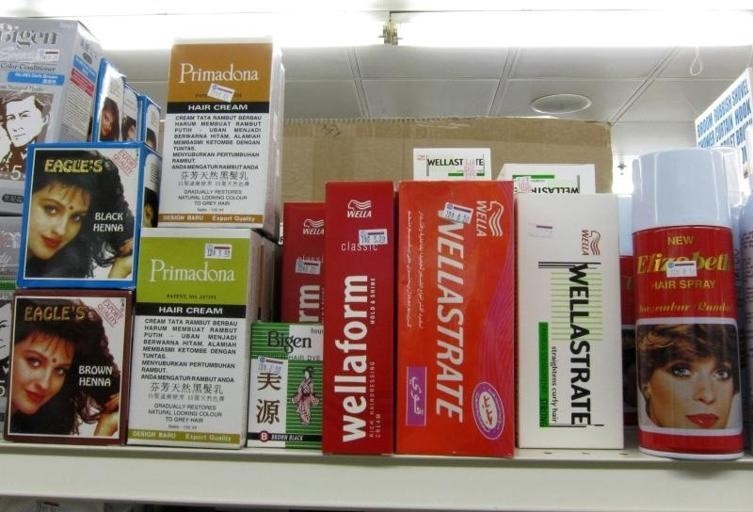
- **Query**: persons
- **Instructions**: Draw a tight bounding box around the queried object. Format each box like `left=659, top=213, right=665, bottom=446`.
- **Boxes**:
left=124, top=111, right=138, bottom=147
left=29, top=151, right=133, bottom=278
left=141, top=189, right=159, bottom=228
left=13, top=301, right=118, bottom=435
left=637, top=322, right=740, bottom=433
left=98, top=96, right=121, bottom=143
left=0, top=296, right=14, bottom=431
left=290, top=365, right=319, bottom=429
left=2, top=90, right=49, bottom=172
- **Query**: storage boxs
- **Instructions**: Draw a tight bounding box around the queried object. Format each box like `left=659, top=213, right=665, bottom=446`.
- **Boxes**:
left=265, top=109, right=612, bottom=203
